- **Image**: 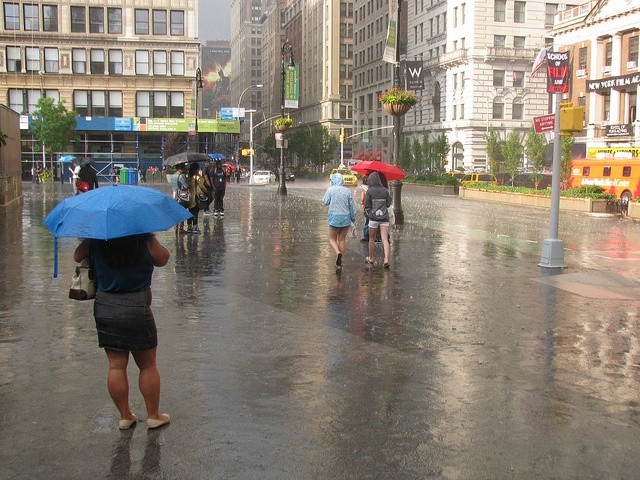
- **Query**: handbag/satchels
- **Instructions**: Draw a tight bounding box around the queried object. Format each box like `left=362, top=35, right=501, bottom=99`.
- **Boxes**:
left=195, top=174, right=209, bottom=210
left=68, top=240, right=97, bottom=300
left=175, top=173, right=192, bottom=208
left=76, top=167, right=89, bottom=192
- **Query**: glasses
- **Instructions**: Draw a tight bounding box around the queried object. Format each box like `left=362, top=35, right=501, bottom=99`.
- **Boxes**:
left=217, top=164, right=222, bottom=165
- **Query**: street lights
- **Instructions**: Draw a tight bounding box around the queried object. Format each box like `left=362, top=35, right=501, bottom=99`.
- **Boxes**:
left=238, top=83, right=264, bottom=114
left=196, top=68, right=203, bottom=158
left=277, top=42, right=295, bottom=194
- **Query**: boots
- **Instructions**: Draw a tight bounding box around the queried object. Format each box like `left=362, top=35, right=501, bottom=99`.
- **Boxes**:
left=187, top=224, right=193, bottom=233
left=192, top=224, right=201, bottom=234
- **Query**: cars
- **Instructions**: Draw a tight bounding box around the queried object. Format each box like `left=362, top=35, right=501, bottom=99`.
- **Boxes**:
left=278, top=169, right=295, bottom=183
left=458, top=173, right=497, bottom=189
left=448, top=173, right=465, bottom=182
left=250, top=170, right=275, bottom=185
left=329, top=168, right=358, bottom=188
left=507, top=174, right=552, bottom=190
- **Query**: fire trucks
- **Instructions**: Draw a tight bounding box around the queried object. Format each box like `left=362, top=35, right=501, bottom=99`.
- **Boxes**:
left=567, top=158, right=640, bottom=205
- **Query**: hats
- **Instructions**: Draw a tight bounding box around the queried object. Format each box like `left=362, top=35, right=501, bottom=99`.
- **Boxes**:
left=187, top=162, right=200, bottom=170
left=216, top=160, right=223, bottom=164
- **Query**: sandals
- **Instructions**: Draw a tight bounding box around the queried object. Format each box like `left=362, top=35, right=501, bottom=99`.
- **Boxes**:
left=365, top=256, right=377, bottom=266
left=383, top=263, right=390, bottom=268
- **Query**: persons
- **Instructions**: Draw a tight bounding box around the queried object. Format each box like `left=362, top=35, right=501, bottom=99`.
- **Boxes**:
left=78, top=157, right=99, bottom=192
left=322, top=173, right=359, bottom=274
left=109, top=167, right=120, bottom=183
left=68, top=159, right=81, bottom=193
left=186, top=162, right=208, bottom=234
left=234, top=165, right=242, bottom=183
left=170, top=162, right=189, bottom=234
left=146, top=164, right=160, bottom=173
left=138, top=166, right=144, bottom=183
left=73, top=232, right=170, bottom=430
left=202, top=159, right=215, bottom=215
left=210, top=160, right=226, bottom=217
left=361, top=168, right=392, bottom=268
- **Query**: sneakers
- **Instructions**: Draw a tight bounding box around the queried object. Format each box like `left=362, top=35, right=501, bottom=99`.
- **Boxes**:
left=219, top=212, right=224, bottom=216
left=119, top=413, right=137, bottom=430
left=360, top=237, right=366, bottom=241
left=337, top=266, right=342, bottom=270
left=213, top=210, right=219, bottom=216
left=146, top=413, right=170, bottom=429
left=336, top=252, right=342, bottom=266
left=203, top=212, right=212, bottom=217
left=207, top=210, right=214, bottom=213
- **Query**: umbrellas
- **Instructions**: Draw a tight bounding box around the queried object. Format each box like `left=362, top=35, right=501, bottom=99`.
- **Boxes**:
left=162, top=151, right=210, bottom=167
left=351, top=159, right=406, bottom=181
left=58, top=155, right=77, bottom=164
left=42, top=183, right=194, bottom=242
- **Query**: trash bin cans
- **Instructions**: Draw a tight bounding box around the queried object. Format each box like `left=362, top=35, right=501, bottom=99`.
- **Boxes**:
left=128, top=167, right=138, bottom=185
left=120, top=167, right=128, bottom=185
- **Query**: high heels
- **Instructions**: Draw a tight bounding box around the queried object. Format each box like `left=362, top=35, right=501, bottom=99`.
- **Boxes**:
left=175, top=229, right=178, bottom=234
left=179, top=228, right=187, bottom=234
